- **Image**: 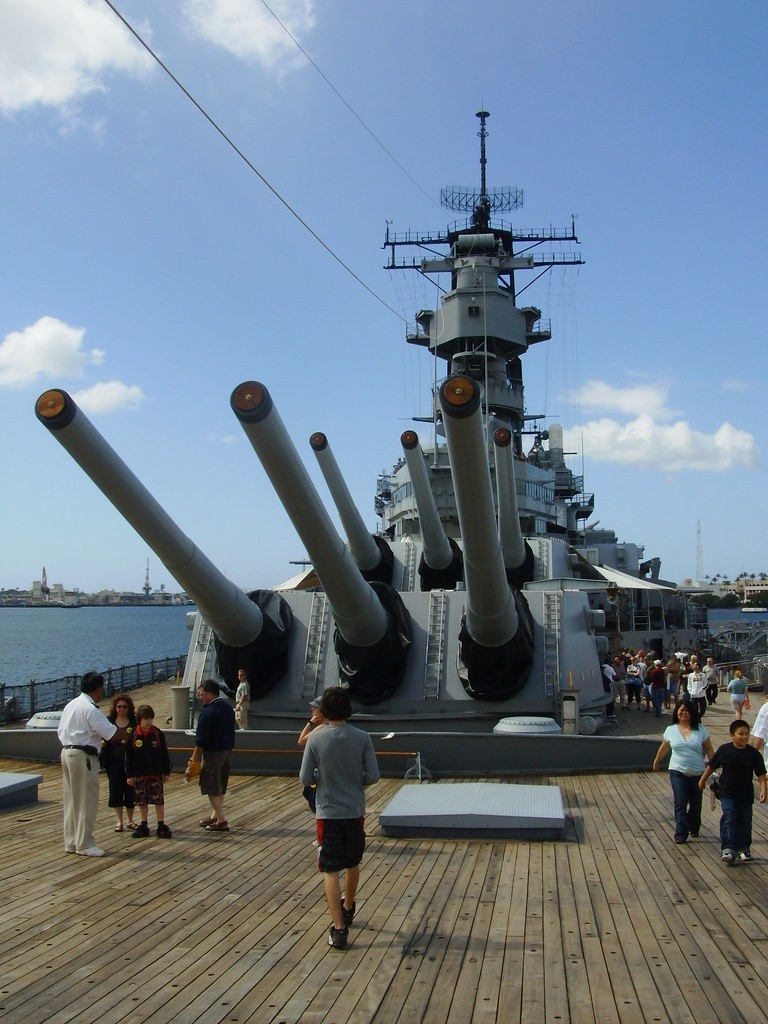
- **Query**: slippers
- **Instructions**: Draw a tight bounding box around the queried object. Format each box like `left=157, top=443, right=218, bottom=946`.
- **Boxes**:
left=127, top=823, right=139, bottom=830
left=114, top=825, right=123, bottom=832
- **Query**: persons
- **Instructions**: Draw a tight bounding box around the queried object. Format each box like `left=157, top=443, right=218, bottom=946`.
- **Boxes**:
left=698, top=720, right=767, bottom=863
left=298, top=696, right=323, bottom=845
left=100, top=695, right=140, bottom=831
left=687, top=662, right=709, bottom=723
left=299, top=687, right=380, bottom=948
left=126, top=705, right=172, bottom=838
left=727, top=670, right=750, bottom=720
left=57, top=671, right=126, bottom=857
left=653, top=700, right=715, bottom=844
left=233, top=669, right=250, bottom=730
left=186, top=680, right=235, bottom=831
left=600, top=647, right=720, bottom=717
left=750, top=702, right=768, bottom=781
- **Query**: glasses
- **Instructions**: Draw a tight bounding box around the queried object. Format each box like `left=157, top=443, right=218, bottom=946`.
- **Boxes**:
left=116, top=705, right=127, bottom=709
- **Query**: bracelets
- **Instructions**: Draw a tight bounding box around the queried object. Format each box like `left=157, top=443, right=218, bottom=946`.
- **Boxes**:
left=309, top=720, right=313, bottom=725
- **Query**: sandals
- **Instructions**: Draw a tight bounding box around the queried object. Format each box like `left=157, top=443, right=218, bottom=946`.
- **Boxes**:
left=206, top=821, right=229, bottom=831
left=199, top=817, right=217, bottom=826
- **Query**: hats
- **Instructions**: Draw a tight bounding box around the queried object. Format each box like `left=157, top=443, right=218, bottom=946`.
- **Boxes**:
left=308, top=696, right=322, bottom=709
left=655, top=660, right=661, bottom=666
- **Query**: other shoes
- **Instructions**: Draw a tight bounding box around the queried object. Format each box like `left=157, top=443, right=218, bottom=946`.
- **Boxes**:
left=65, top=847, right=76, bottom=853
left=312, top=840, right=319, bottom=845
left=76, top=846, right=105, bottom=856
left=157, top=825, right=172, bottom=838
left=606, top=704, right=663, bottom=718
left=676, top=838, right=683, bottom=844
left=690, top=831, right=699, bottom=837
left=132, top=825, right=149, bottom=837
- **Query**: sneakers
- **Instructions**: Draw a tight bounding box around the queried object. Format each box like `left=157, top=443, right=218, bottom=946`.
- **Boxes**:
left=720, top=853, right=732, bottom=864
left=328, top=924, right=349, bottom=947
left=739, top=852, right=752, bottom=861
left=341, top=896, right=356, bottom=926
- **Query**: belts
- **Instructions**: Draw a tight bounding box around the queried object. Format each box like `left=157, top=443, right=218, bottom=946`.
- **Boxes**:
left=63, top=745, right=98, bottom=757
